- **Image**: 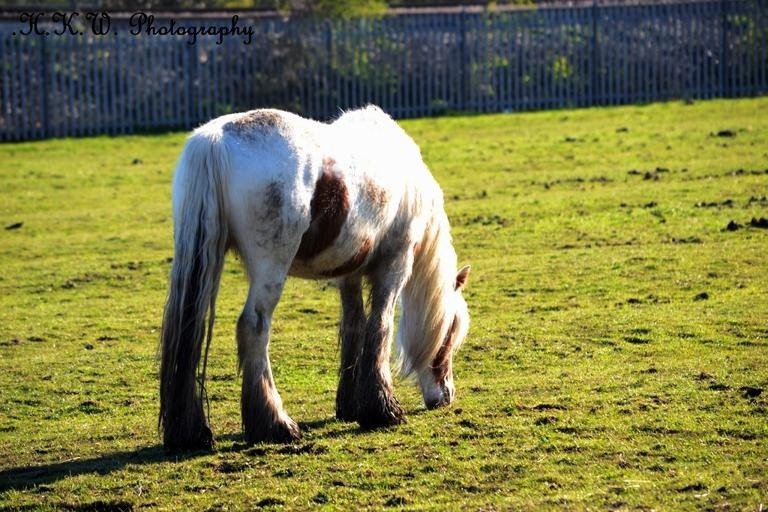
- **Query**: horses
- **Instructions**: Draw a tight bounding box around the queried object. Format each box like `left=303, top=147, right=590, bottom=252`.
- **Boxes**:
left=158, top=103, right=472, bottom=456
